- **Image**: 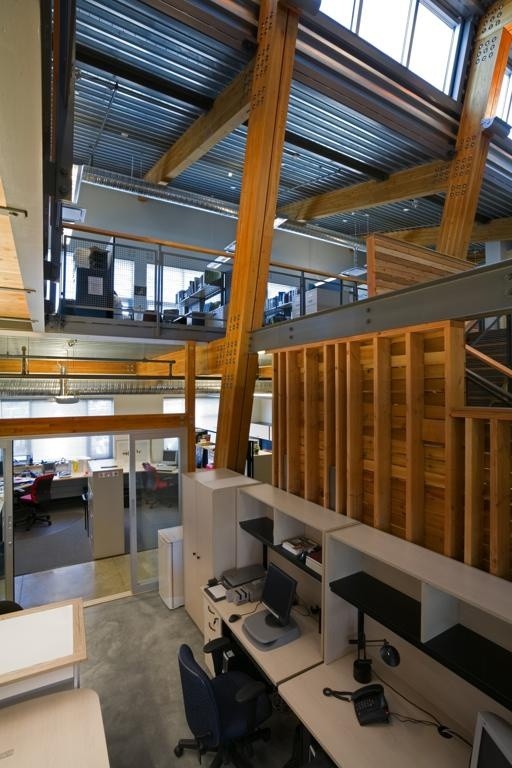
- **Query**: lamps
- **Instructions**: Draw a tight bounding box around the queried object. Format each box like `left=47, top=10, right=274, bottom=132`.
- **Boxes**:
left=483, top=117, right=512, bottom=139
left=348, top=608, right=400, bottom=684
left=54, top=338, right=79, bottom=404
left=342, top=214, right=369, bottom=277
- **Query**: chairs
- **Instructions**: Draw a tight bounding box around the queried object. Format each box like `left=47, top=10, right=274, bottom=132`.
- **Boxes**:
left=175, top=636, right=272, bottom=768
left=19, top=473, right=55, bottom=531
left=0, top=600, right=21, bottom=616
left=143, top=311, right=162, bottom=322
left=163, top=310, right=180, bottom=322
left=142, top=463, right=172, bottom=509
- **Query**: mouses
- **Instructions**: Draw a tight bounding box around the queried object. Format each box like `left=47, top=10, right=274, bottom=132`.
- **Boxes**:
left=229, top=614, right=240, bottom=622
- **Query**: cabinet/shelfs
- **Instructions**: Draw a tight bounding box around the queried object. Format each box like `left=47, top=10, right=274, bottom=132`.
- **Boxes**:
left=277, top=524, right=512, bottom=767
left=87, top=459, right=125, bottom=560
left=180, top=467, right=361, bottom=688
left=195, top=438, right=275, bottom=484
left=176, top=270, right=343, bottom=327
left=75, top=267, right=108, bottom=317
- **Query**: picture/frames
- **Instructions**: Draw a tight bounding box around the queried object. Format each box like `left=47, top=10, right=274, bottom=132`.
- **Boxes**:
left=116, top=439, right=152, bottom=468
left=134, top=286, right=146, bottom=295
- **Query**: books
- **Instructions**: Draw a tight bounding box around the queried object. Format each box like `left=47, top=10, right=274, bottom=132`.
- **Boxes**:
left=280, top=534, right=322, bottom=576
left=265, top=289, right=298, bottom=311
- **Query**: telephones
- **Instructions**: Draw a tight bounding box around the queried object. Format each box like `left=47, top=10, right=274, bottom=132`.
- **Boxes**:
left=353, top=683, right=390, bottom=725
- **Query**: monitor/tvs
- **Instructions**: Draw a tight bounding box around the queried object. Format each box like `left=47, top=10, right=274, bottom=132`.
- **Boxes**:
left=163, top=450, right=177, bottom=465
left=163, top=309, right=179, bottom=320
left=260, top=564, right=297, bottom=627
left=470, top=710, right=512, bottom=768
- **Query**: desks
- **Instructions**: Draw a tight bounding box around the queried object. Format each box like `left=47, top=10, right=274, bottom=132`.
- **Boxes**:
left=0, top=598, right=112, bottom=767
left=122, top=462, right=182, bottom=502
left=0, top=471, right=88, bottom=513
